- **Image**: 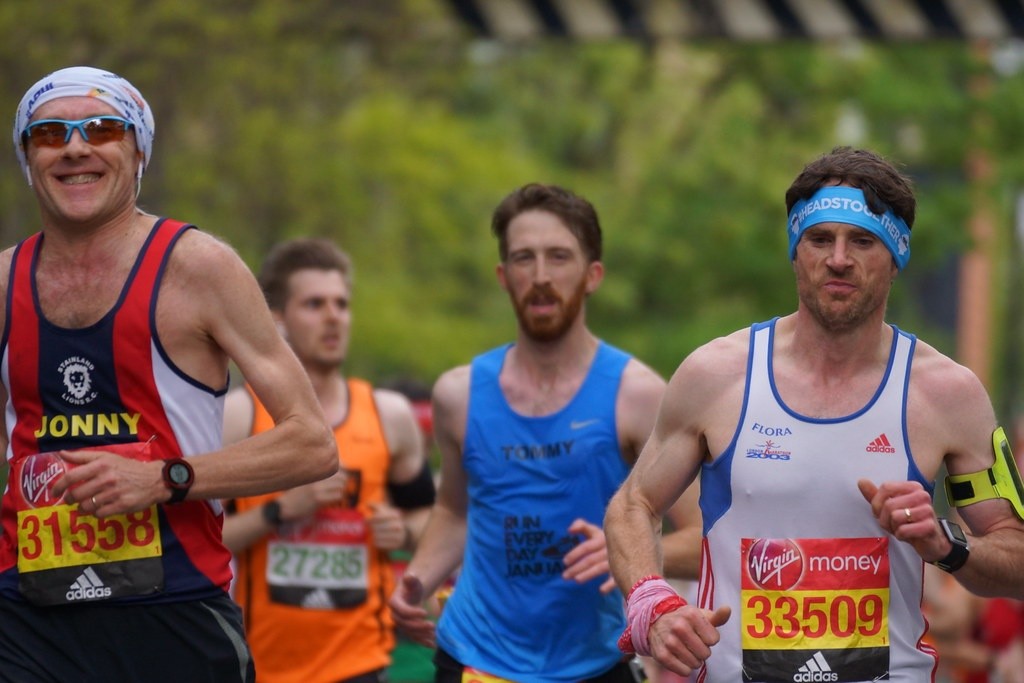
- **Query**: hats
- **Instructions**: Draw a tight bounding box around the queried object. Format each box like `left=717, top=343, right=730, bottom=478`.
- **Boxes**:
left=13, top=66, right=155, bottom=177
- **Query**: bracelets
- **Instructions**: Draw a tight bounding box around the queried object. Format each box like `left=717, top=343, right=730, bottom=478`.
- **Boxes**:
left=400, top=528, right=413, bottom=551
left=617, top=575, right=688, bottom=655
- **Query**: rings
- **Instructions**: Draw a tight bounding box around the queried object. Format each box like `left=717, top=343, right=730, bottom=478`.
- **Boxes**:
left=93, top=496, right=97, bottom=508
left=905, top=508, right=911, bottom=517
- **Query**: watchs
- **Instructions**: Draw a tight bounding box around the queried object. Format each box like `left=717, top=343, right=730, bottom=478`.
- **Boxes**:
left=262, top=500, right=289, bottom=526
left=161, top=457, right=195, bottom=506
left=934, top=518, right=970, bottom=573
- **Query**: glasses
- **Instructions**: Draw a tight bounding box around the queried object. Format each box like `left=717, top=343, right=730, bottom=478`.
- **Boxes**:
left=19, top=116, right=133, bottom=148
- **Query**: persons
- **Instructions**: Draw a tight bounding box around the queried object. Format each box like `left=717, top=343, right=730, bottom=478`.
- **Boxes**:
left=0, top=66, right=339, bottom=683
left=386, top=181, right=704, bottom=683
left=216, top=239, right=462, bottom=683
left=604, top=145, right=1023, bottom=683
